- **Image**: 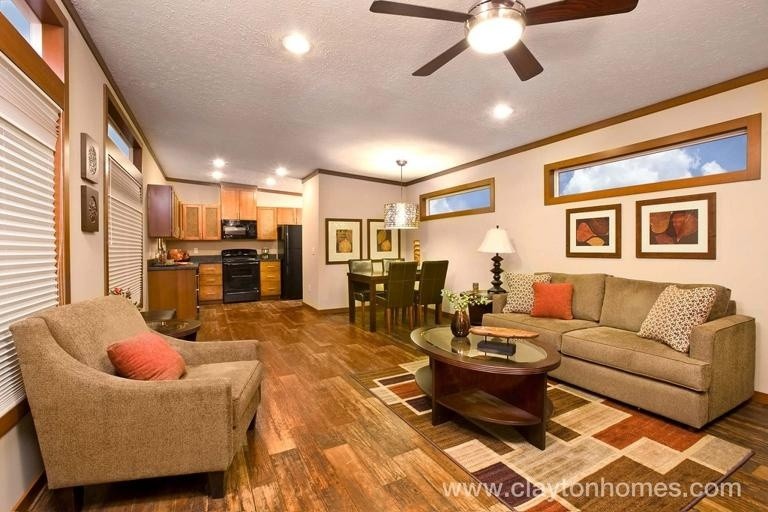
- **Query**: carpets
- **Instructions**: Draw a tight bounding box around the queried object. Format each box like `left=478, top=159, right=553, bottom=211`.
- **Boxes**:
left=343, top=350, right=757, bottom=511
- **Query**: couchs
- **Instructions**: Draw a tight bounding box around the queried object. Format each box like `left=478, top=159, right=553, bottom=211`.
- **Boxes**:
left=474, top=269, right=757, bottom=433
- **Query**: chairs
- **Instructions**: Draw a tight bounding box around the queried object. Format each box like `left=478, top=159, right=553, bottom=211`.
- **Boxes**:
left=344, top=257, right=451, bottom=335
left=7, top=291, right=268, bottom=512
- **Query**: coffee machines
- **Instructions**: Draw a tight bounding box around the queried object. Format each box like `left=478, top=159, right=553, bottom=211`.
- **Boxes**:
left=261, top=246, right=269, bottom=259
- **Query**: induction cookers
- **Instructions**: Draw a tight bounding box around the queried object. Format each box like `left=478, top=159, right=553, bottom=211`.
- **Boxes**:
left=221, top=248, right=260, bottom=262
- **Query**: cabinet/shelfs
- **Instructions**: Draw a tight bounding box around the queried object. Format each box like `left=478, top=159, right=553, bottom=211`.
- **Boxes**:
left=143, top=178, right=303, bottom=324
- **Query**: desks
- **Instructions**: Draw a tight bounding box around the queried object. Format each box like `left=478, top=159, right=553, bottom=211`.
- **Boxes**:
left=135, top=307, right=203, bottom=343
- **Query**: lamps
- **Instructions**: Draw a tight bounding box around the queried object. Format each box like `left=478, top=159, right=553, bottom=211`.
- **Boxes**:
left=463, top=1, right=530, bottom=58
left=382, top=159, right=421, bottom=231
left=477, top=222, right=518, bottom=295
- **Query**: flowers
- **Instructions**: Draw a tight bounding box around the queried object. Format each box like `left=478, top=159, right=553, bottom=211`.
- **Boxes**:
left=108, top=286, right=142, bottom=311
left=438, top=287, right=493, bottom=312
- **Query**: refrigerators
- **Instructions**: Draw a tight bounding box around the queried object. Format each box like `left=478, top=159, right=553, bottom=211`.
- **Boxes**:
left=277, top=224, right=304, bottom=300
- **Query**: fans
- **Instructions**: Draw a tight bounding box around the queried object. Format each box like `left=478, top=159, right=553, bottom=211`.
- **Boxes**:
left=360, top=0, right=639, bottom=87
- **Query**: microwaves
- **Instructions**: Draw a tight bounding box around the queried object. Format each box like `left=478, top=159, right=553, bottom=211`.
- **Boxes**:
left=221, top=219, right=258, bottom=239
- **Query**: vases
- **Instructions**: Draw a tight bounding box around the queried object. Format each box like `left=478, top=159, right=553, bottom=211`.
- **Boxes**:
left=449, top=308, right=471, bottom=339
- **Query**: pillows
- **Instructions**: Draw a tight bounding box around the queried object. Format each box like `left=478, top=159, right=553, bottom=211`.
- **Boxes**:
left=528, top=279, right=575, bottom=320
left=104, top=330, right=188, bottom=383
left=500, top=271, right=553, bottom=315
left=634, top=281, right=720, bottom=354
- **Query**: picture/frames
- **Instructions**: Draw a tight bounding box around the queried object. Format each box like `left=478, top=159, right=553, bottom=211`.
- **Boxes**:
left=323, top=216, right=364, bottom=265
left=366, top=217, right=401, bottom=263
left=564, top=203, right=625, bottom=259
left=632, top=190, right=720, bottom=261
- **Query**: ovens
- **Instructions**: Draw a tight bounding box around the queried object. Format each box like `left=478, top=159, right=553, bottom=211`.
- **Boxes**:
left=222, top=262, right=260, bottom=294
